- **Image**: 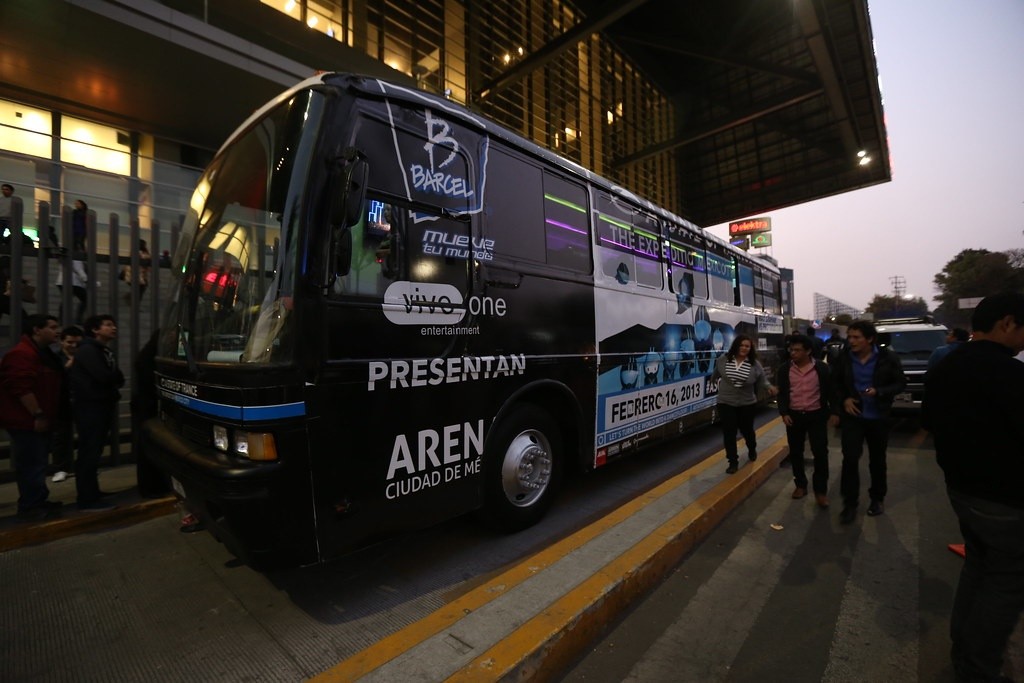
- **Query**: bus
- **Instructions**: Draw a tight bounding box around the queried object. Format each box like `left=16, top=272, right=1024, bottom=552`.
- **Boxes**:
left=143, top=71, right=786, bottom=562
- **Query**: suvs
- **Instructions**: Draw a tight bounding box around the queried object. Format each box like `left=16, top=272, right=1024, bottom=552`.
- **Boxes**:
left=876, top=315, right=954, bottom=417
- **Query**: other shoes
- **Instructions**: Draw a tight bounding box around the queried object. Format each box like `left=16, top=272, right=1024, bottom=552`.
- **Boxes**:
left=748, top=447, right=756, bottom=461
left=99, top=492, right=120, bottom=499
left=792, top=487, right=807, bottom=498
left=841, top=503, right=856, bottom=522
left=51, top=471, right=68, bottom=482
left=33, top=501, right=63, bottom=509
left=814, top=489, right=829, bottom=508
left=725, top=461, right=738, bottom=474
left=867, top=497, right=885, bottom=515
left=17, top=506, right=48, bottom=521
left=74, top=500, right=117, bottom=512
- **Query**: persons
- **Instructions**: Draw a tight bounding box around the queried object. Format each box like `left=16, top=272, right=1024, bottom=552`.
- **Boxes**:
left=131, top=328, right=178, bottom=496
left=711, top=333, right=777, bottom=474
left=777, top=335, right=840, bottom=508
left=0, top=256, right=27, bottom=319
left=73, top=314, right=124, bottom=511
left=832, top=320, right=908, bottom=523
left=73, top=198, right=88, bottom=251
left=125, top=239, right=151, bottom=306
left=792, top=327, right=827, bottom=360
left=37, top=200, right=58, bottom=247
left=928, top=328, right=969, bottom=366
left=52, top=327, right=84, bottom=482
left=825, top=329, right=846, bottom=363
left=0, top=184, right=32, bottom=243
left=0, top=313, right=64, bottom=520
left=924, top=293, right=1024, bottom=683
left=55, top=240, right=101, bottom=323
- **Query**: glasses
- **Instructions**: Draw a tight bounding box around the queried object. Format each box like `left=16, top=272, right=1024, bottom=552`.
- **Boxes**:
left=787, top=347, right=806, bottom=353
